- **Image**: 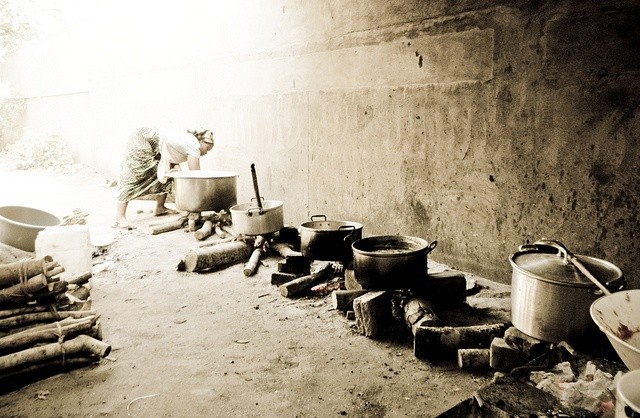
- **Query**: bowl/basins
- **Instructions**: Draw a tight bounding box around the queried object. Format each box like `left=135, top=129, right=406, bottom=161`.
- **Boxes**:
left=0, top=205, right=64, bottom=251
left=590, top=289, right=640, bottom=370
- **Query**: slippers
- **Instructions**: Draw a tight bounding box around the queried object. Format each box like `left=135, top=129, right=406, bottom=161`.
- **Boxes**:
left=153, top=209, right=180, bottom=215
left=112, top=221, right=136, bottom=230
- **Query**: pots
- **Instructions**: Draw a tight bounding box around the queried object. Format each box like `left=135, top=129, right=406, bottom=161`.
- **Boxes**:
left=167, top=170, right=239, bottom=213
left=508, top=245, right=624, bottom=350
left=344, top=232, right=437, bottom=291
left=230, top=197, right=284, bottom=236
left=300, top=214, right=363, bottom=261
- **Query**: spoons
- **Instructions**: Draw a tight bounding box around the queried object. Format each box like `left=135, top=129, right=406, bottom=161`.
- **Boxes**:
left=534, top=238, right=612, bottom=295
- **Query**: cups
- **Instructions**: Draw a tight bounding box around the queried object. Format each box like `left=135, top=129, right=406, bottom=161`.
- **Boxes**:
left=615, top=371, right=639, bottom=416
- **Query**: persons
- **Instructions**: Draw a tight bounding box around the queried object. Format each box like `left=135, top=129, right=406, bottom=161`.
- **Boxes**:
left=113, top=126, right=214, bottom=230
